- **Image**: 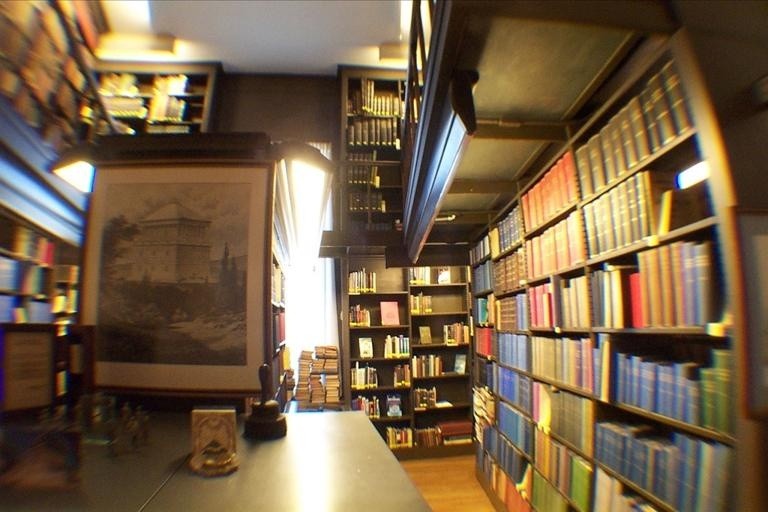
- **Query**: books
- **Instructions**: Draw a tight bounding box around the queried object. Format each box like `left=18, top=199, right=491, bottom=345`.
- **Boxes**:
left=467, top=63, right=734, bottom=512
left=294, top=346, right=343, bottom=409
left=347, top=77, right=423, bottom=213
left=0, top=0, right=186, bottom=328
left=348, top=265, right=471, bottom=448
left=272, top=265, right=294, bottom=413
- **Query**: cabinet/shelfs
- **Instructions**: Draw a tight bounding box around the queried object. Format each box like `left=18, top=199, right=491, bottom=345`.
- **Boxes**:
left=328, top=1, right=767, bottom=509
left=1, top=0, right=293, bottom=415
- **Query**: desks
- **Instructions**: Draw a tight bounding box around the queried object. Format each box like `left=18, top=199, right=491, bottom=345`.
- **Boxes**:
left=2, top=406, right=437, bottom=511
